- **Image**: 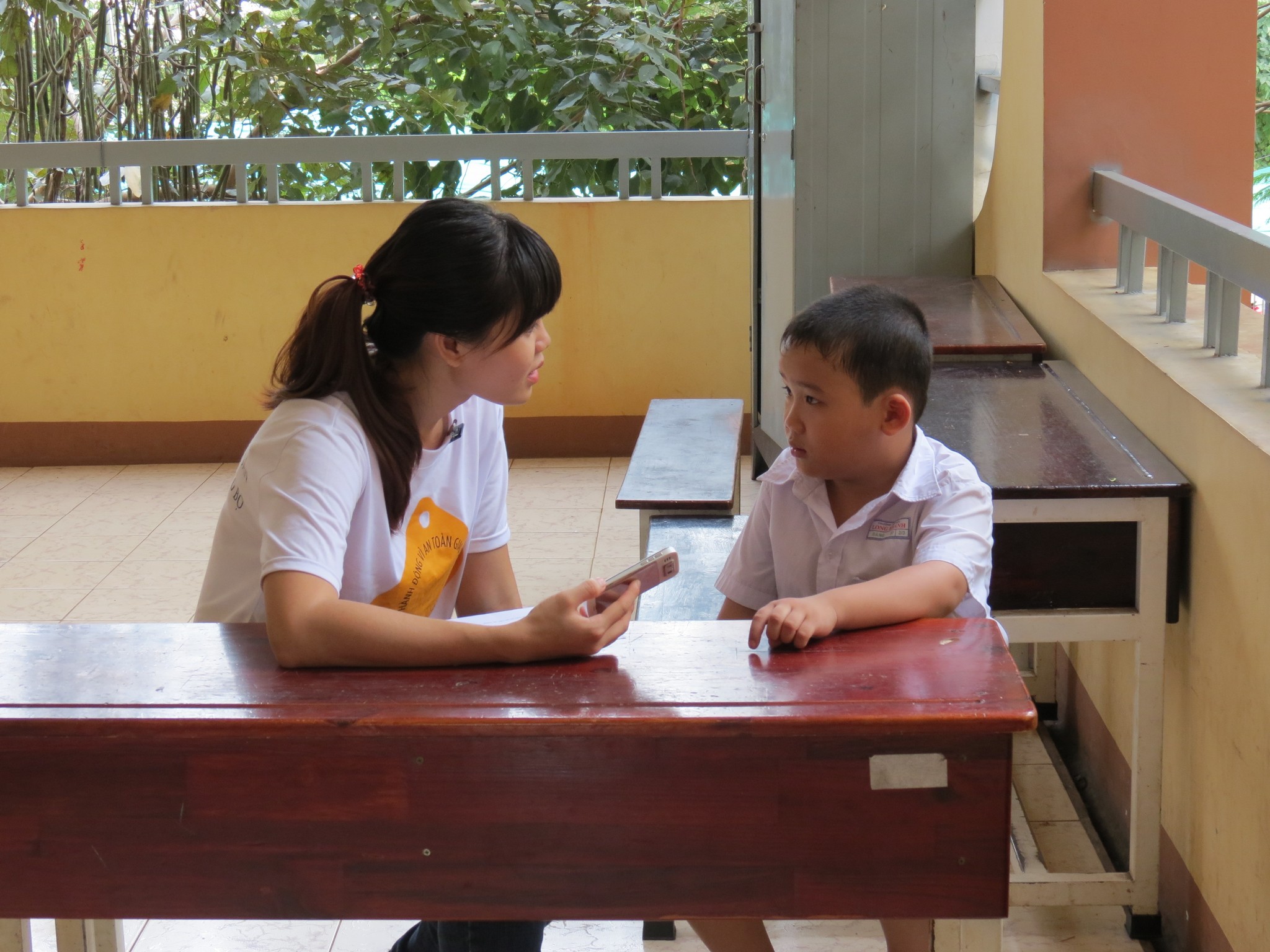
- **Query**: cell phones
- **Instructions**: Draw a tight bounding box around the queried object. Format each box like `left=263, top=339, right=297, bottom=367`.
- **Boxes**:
left=587, top=547, right=680, bottom=617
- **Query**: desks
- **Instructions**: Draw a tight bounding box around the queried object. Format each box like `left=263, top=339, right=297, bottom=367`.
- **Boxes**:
left=828, top=273, right=1046, bottom=366
left=907, top=364, right=1195, bottom=942
left=0, top=623, right=1040, bottom=952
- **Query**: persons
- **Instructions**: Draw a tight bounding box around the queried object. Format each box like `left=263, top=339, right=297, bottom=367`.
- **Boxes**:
left=686, top=284, right=993, bottom=952
left=194, top=198, right=644, bottom=952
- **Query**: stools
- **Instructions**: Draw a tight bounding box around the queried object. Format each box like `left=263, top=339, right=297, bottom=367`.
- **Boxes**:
left=617, top=398, right=742, bottom=588
